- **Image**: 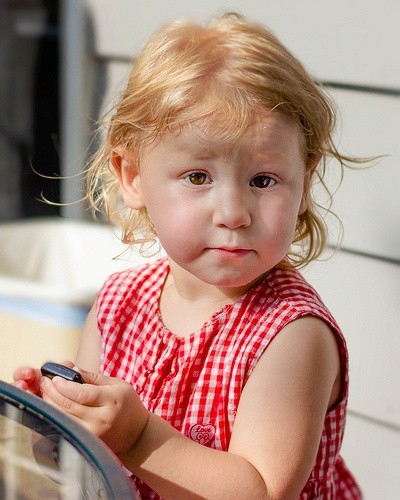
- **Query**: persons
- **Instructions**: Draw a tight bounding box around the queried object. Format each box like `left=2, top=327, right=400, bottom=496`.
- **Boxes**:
left=10, top=12, right=363, bottom=500
